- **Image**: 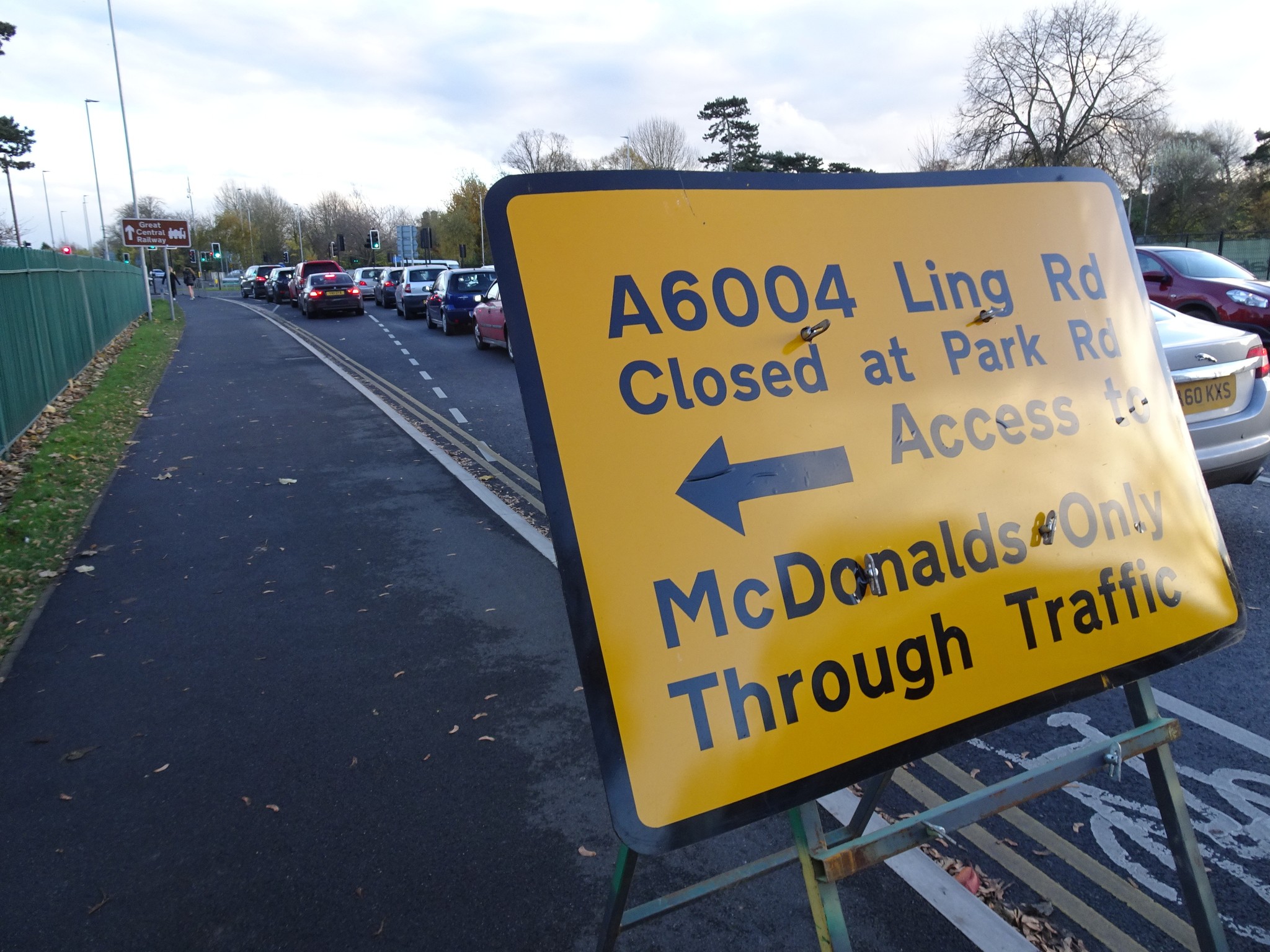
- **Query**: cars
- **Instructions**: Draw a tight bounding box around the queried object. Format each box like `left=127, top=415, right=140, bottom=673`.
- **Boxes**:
left=424, top=266, right=495, bottom=335
left=374, top=267, right=406, bottom=309
left=1126, top=244, right=1270, bottom=348
left=264, top=266, right=295, bottom=303
left=228, top=269, right=245, bottom=279
left=1115, top=297, right=1270, bottom=489
left=472, top=276, right=516, bottom=362
left=299, top=272, right=365, bottom=318
left=149, top=269, right=165, bottom=278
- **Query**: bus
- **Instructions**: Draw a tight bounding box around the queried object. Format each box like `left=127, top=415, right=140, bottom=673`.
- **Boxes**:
left=401, top=259, right=460, bottom=271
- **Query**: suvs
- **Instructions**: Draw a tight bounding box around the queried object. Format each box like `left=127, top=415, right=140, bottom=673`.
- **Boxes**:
left=353, top=267, right=386, bottom=300
left=287, top=260, right=343, bottom=309
left=396, top=264, right=448, bottom=319
left=241, top=264, right=281, bottom=298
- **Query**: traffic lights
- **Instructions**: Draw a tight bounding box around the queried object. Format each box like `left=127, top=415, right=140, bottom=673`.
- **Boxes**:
left=211, top=242, right=222, bottom=259
left=338, top=233, right=345, bottom=251
left=206, top=252, right=210, bottom=262
left=210, top=251, right=213, bottom=260
left=370, top=230, right=380, bottom=249
left=350, top=258, right=358, bottom=264
left=123, top=253, right=130, bottom=264
left=189, top=249, right=196, bottom=263
left=62, top=247, right=71, bottom=254
left=200, top=251, right=206, bottom=262
left=283, top=252, right=289, bottom=263
left=146, top=247, right=158, bottom=250
left=331, top=242, right=337, bottom=258
left=364, top=233, right=371, bottom=249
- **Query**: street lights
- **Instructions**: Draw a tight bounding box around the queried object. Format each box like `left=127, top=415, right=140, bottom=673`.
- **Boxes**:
left=82, top=193, right=94, bottom=259
left=84, top=97, right=110, bottom=258
left=62, top=210, right=67, bottom=247
left=237, top=187, right=247, bottom=263
left=42, top=168, right=56, bottom=250
left=292, top=202, right=304, bottom=262
